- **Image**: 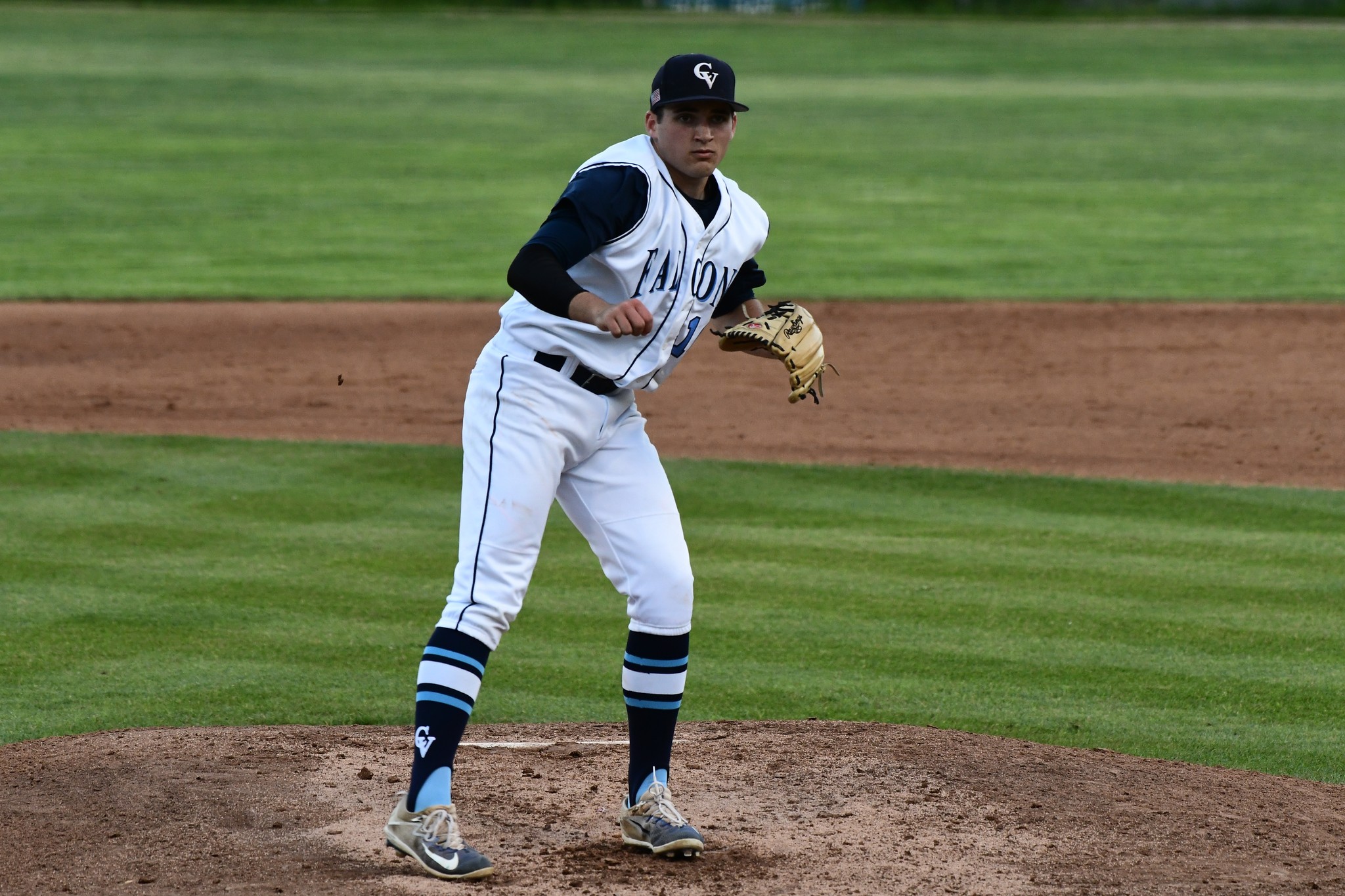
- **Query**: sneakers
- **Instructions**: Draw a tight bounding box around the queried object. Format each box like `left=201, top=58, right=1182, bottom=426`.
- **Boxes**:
left=621, top=765, right=704, bottom=858
left=383, top=798, right=494, bottom=879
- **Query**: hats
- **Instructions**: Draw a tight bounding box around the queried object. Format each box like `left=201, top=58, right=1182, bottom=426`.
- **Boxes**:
left=650, top=53, right=750, bottom=113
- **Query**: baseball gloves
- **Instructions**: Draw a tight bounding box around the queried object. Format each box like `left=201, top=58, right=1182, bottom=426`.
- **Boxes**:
left=718, top=302, right=824, bottom=405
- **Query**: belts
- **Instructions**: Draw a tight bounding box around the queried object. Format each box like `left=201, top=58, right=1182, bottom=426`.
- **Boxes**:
left=534, top=350, right=615, bottom=395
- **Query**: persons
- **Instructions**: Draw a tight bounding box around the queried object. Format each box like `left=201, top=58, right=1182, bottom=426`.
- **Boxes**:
left=381, top=54, right=828, bottom=880
